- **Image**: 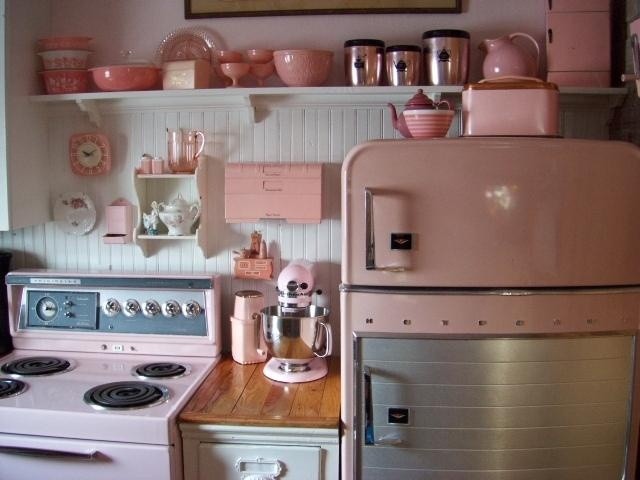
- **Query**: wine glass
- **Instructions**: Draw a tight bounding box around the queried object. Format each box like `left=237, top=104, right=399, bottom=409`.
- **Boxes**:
left=215, top=49, right=275, bottom=88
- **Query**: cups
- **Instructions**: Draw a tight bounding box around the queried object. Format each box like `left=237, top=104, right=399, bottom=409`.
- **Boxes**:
left=261, top=305, right=331, bottom=370
left=166, top=128, right=205, bottom=173
left=344, top=29, right=470, bottom=87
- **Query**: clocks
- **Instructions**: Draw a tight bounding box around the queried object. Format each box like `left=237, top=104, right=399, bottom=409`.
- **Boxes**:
left=68, top=132, right=112, bottom=176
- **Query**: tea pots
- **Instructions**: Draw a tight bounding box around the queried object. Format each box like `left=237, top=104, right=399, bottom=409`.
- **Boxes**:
left=387, top=90, right=452, bottom=140
left=158, top=192, right=201, bottom=236
left=478, top=32, right=540, bottom=78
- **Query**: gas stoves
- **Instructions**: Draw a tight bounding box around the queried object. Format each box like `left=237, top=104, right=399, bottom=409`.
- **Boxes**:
left=1, top=353, right=216, bottom=418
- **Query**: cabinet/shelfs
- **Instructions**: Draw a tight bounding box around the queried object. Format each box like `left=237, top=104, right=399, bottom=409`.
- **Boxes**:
left=130, top=154, right=210, bottom=259
left=544, top=0, right=611, bottom=89
left=177, top=353, right=342, bottom=480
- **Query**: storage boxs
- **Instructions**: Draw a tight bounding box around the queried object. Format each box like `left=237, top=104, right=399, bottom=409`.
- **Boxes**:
left=457, top=74, right=563, bottom=138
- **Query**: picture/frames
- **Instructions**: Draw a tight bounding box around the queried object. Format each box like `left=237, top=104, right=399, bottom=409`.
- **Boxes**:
left=182, top=0, right=462, bottom=19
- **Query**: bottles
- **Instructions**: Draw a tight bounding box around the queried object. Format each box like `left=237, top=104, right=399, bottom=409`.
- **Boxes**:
left=231, top=290, right=267, bottom=366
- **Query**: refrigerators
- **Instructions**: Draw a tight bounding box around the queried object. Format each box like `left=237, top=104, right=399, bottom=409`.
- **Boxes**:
left=339, top=137, right=640, bottom=480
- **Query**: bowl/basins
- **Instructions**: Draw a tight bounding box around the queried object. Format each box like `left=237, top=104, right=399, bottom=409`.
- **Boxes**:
left=402, top=108, right=455, bottom=139
left=88, top=64, right=162, bottom=91
left=36, top=36, right=94, bottom=93
left=273, top=49, right=334, bottom=87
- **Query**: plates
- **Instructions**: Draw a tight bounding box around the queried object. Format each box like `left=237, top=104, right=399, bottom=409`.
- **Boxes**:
left=53, top=190, right=97, bottom=238
left=153, top=27, right=222, bottom=83
